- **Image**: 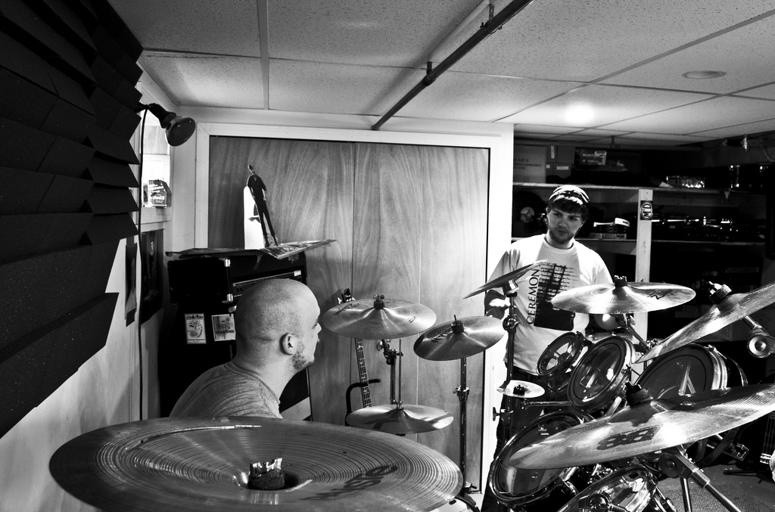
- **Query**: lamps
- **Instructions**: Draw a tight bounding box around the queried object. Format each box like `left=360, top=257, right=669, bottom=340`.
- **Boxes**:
left=137, top=102, right=196, bottom=422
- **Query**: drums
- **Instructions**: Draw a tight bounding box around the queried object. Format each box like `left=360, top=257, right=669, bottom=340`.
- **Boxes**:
left=566, top=335, right=646, bottom=410
left=558, top=464, right=675, bottom=512
left=620, top=343, right=748, bottom=468
left=487, top=410, right=598, bottom=512
left=536, top=333, right=593, bottom=392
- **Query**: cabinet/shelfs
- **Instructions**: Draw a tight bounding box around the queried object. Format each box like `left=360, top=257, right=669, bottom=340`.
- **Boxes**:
left=648, top=246, right=765, bottom=342
left=208, top=135, right=353, bottom=427
left=353, top=142, right=489, bottom=493
left=586, top=250, right=636, bottom=334
left=513, top=136, right=774, bottom=191
left=511, top=185, right=638, bottom=239
left=651, top=190, right=775, bottom=242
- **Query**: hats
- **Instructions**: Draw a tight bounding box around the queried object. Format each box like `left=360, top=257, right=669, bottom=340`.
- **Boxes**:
left=549, top=185, right=590, bottom=216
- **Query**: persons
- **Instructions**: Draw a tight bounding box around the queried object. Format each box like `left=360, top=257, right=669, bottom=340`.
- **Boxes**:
left=480, top=185, right=637, bottom=512
left=248, top=165, right=280, bottom=247
left=170, top=278, right=321, bottom=421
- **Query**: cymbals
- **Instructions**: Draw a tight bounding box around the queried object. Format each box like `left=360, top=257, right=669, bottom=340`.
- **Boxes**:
left=48, top=415, right=463, bottom=512
left=551, top=282, right=695, bottom=314
left=413, top=317, right=504, bottom=361
left=322, top=299, right=437, bottom=340
left=463, top=259, right=548, bottom=300
left=501, top=381, right=545, bottom=398
left=636, top=284, right=775, bottom=362
left=347, top=404, right=454, bottom=435
left=509, top=387, right=775, bottom=470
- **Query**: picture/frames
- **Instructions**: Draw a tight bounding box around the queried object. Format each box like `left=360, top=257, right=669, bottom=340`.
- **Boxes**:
left=640, top=199, right=653, bottom=220
left=139, top=228, right=165, bottom=325
